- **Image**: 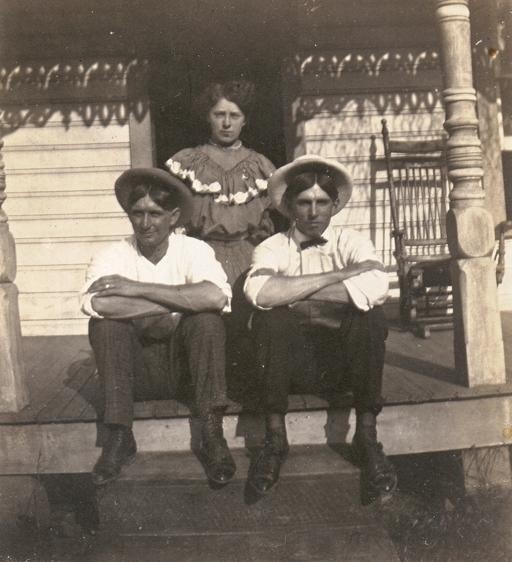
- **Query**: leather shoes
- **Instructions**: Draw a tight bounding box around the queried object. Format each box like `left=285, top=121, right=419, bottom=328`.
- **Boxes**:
left=199, top=436, right=235, bottom=483
left=352, top=434, right=399, bottom=496
left=90, top=430, right=137, bottom=483
left=251, top=441, right=289, bottom=494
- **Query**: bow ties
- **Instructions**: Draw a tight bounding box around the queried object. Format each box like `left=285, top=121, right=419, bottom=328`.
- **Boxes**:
left=300, top=238, right=327, bottom=251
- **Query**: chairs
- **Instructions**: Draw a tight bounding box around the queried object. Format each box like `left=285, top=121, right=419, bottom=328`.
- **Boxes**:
left=380, top=119, right=511, bottom=339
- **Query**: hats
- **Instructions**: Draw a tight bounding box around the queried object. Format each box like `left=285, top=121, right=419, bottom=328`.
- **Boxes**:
left=268, top=154, right=353, bottom=218
left=116, top=167, right=195, bottom=228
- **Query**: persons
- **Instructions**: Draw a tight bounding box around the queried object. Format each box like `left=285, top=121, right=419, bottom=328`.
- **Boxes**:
left=243, top=155, right=396, bottom=495
left=163, top=82, right=278, bottom=331
left=78, top=167, right=236, bottom=484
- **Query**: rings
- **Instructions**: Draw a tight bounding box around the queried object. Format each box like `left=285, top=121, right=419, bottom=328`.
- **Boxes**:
left=105, top=283, right=109, bottom=288
